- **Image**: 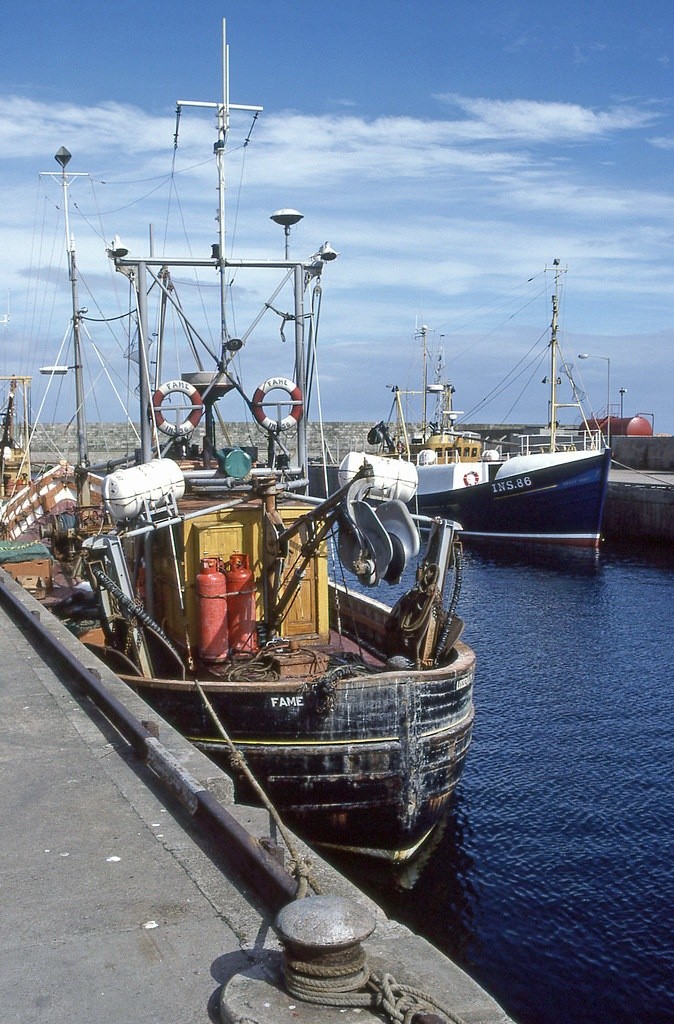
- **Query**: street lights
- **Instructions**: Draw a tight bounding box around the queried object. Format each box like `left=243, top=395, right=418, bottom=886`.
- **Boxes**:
left=578, top=353, right=610, bottom=448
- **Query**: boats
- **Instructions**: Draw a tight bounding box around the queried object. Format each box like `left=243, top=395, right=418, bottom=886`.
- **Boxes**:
left=366, top=255, right=614, bottom=550
left=0, top=17, right=478, bottom=901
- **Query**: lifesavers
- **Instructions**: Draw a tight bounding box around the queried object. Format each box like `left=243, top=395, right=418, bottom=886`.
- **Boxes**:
left=464, top=471, right=479, bottom=487
left=252, top=377, right=305, bottom=434
left=149, top=381, right=202, bottom=437
